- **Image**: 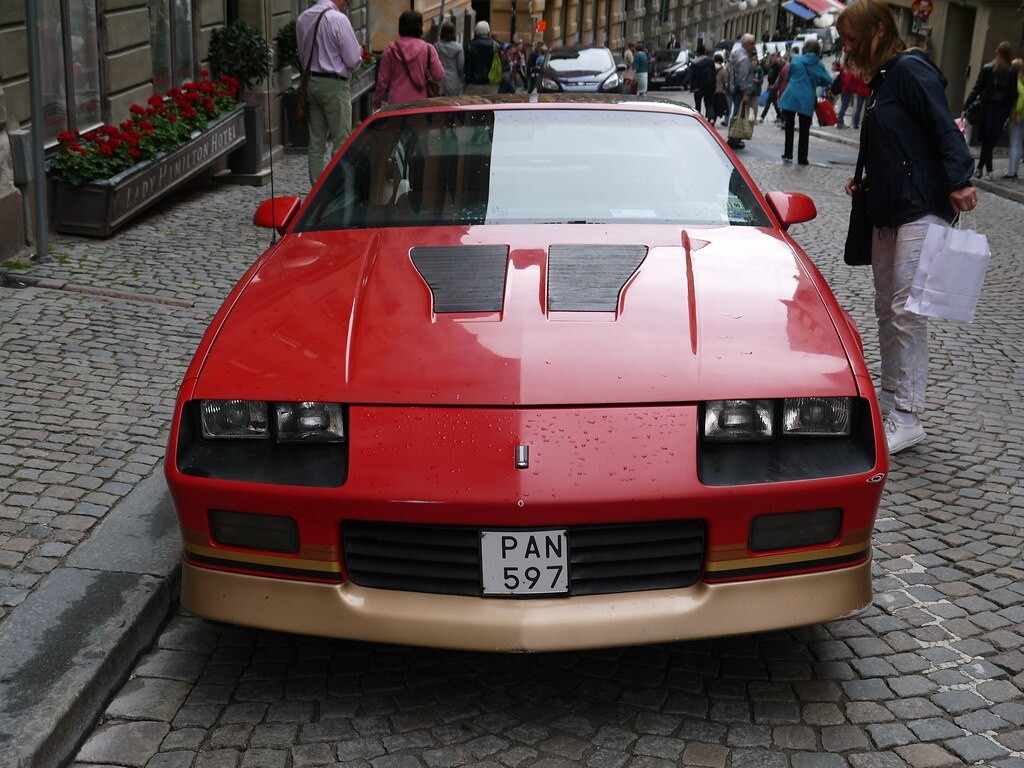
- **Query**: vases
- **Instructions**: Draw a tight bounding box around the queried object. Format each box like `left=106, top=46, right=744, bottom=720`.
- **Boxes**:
left=349, top=66, right=376, bottom=104
left=51, top=102, right=246, bottom=237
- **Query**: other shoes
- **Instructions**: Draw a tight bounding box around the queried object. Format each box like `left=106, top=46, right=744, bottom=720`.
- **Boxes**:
left=781, top=154, right=793, bottom=160
left=721, top=121, right=728, bottom=126
left=1000, top=171, right=1018, bottom=181
left=773, top=118, right=796, bottom=132
left=727, top=139, right=745, bottom=149
left=760, top=117, right=764, bottom=123
left=837, top=124, right=850, bottom=129
left=853, top=126, right=859, bottom=129
left=798, top=160, right=809, bottom=165
left=973, top=167, right=983, bottom=179
left=709, top=119, right=715, bottom=127
left=984, top=173, right=993, bottom=182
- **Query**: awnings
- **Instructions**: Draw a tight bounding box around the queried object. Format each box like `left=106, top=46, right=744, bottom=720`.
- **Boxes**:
left=781, top=0, right=847, bottom=21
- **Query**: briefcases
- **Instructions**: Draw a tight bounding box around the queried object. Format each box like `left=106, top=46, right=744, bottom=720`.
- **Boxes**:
left=713, top=90, right=730, bottom=118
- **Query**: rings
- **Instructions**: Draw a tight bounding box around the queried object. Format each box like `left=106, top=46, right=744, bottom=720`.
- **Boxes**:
left=972, top=200, right=974, bottom=204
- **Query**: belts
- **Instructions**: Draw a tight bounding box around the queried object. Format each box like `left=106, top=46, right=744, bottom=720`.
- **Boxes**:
left=310, top=72, right=347, bottom=81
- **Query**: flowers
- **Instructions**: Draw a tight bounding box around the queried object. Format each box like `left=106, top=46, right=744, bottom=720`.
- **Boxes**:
left=47, top=70, right=242, bottom=184
left=348, top=45, right=377, bottom=82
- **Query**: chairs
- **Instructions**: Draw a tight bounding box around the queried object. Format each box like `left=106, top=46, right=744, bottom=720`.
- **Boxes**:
left=386, top=151, right=491, bottom=222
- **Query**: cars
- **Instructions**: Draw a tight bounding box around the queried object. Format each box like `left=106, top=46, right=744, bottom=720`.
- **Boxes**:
left=531, top=45, right=627, bottom=93
left=707, top=39, right=792, bottom=65
left=163, top=92, right=892, bottom=654
left=650, top=48, right=698, bottom=91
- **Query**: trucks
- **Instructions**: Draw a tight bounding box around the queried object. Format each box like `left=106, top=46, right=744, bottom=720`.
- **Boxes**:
left=793, top=27, right=832, bottom=59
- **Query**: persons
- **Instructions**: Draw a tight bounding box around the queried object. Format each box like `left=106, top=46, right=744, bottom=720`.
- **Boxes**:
left=961, top=41, right=1024, bottom=179
left=836, top=0, right=980, bottom=454
left=827, top=61, right=869, bottom=129
left=761, top=30, right=779, bottom=41
left=432, top=22, right=465, bottom=96
left=296, top=0, right=362, bottom=186
left=491, top=33, right=548, bottom=93
left=372, top=10, right=446, bottom=111
left=622, top=42, right=657, bottom=96
left=727, top=33, right=755, bottom=149
left=464, top=21, right=502, bottom=96
left=779, top=39, right=834, bottom=165
left=689, top=45, right=799, bottom=127
left=666, top=33, right=681, bottom=49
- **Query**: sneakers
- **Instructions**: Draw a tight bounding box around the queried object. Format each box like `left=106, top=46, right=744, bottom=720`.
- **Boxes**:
left=876, top=393, right=896, bottom=421
left=882, top=410, right=927, bottom=455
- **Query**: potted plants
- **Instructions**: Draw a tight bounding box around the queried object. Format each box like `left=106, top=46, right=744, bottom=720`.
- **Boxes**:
left=208, top=19, right=276, bottom=174
left=272, top=20, right=311, bottom=148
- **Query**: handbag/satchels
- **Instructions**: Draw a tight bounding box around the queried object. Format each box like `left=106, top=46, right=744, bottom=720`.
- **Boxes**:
left=954, top=117, right=967, bottom=135
left=295, top=76, right=308, bottom=122
left=843, top=188, right=874, bottom=266
left=623, top=78, right=638, bottom=96
left=965, top=98, right=986, bottom=125
left=425, top=42, right=440, bottom=97
left=814, top=98, right=838, bottom=127
left=487, top=39, right=503, bottom=86
left=727, top=99, right=755, bottom=140
left=902, top=208, right=992, bottom=325
